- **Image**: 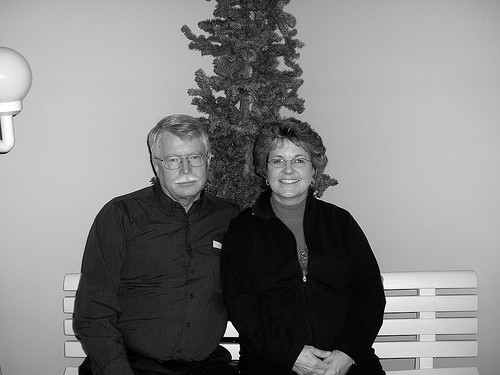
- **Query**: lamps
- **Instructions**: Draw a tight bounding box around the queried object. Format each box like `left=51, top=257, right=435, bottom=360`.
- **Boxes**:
left=0, top=47, right=33, bottom=153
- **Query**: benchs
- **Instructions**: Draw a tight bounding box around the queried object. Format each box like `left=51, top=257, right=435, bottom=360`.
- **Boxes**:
left=63, top=271, right=479, bottom=375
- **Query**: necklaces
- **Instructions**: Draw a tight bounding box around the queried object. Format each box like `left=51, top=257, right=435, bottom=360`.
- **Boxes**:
left=296, top=245, right=308, bottom=264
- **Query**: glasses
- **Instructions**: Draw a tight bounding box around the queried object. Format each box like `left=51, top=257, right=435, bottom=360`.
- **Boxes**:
left=268, top=157, right=312, bottom=167
left=156, top=153, right=208, bottom=170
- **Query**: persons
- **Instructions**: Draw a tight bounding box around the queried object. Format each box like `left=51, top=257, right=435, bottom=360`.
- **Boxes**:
left=72, top=114, right=244, bottom=375
left=219, top=117, right=388, bottom=375
